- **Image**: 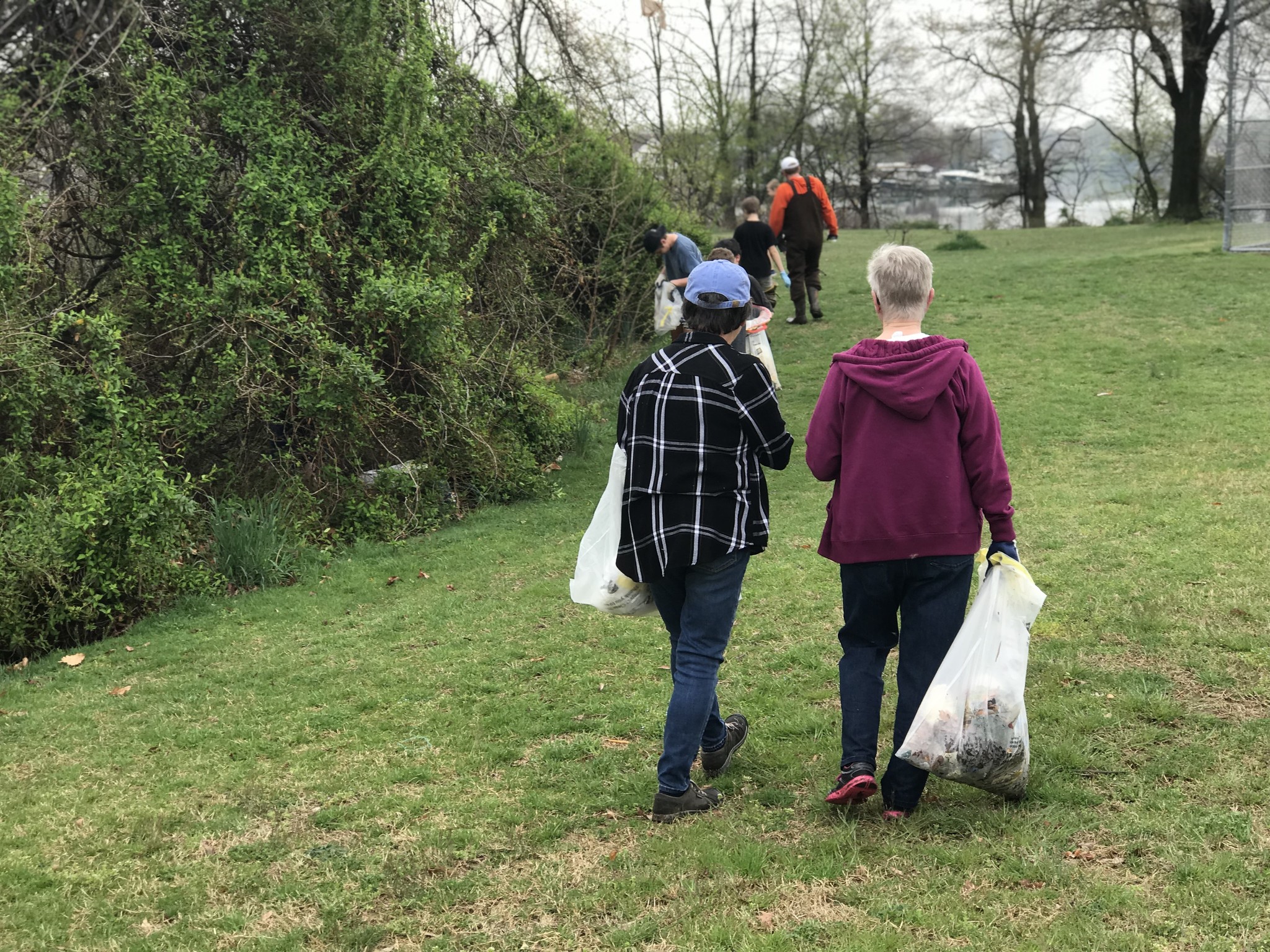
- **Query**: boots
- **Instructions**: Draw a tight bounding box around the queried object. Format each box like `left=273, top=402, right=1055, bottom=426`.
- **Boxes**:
left=786, top=289, right=807, bottom=324
left=807, top=287, right=824, bottom=319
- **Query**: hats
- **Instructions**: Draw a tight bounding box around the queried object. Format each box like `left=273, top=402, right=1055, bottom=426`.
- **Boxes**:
left=780, top=156, right=800, bottom=171
left=684, top=259, right=751, bottom=309
left=644, top=225, right=666, bottom=255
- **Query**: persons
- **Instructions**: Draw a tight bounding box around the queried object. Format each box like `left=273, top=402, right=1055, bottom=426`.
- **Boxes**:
left=768, top=156, right=840, bottom=325
left=805, top=242, right=1021, bottom=820
left=642, top=179, right=792, bottom=353
left=617, top=260, right=794, bottom=824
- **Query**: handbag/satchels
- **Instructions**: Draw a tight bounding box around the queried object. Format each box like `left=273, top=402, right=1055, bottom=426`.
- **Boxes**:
left=894, top=548, right=1048, bottom=800
left=569, top=442, right=660, bottom=617
left=653, top=281, right=683, bottom=336
left=745, top=304, right=783, bottom=391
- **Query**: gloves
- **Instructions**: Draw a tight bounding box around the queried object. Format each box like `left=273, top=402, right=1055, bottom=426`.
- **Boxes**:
left=827, top=234, right=838, bottom=241
left=780, top=272, right=791, bottom=288
left=983, top=539, right=1021, bottom=583
left=655, top=273, right=666, bottom=288
left=667, top=282, right=677, bottom=303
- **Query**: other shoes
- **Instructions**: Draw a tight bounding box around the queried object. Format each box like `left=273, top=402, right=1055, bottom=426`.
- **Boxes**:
left=824, top=761, right=878, bottom=805
left=882, top=803, right=912, bottom=821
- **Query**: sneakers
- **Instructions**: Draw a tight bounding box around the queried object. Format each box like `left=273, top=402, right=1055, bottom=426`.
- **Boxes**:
left=700, top=713, right=749, bottom=779
left=651, top=779, right=723, bottom=824
